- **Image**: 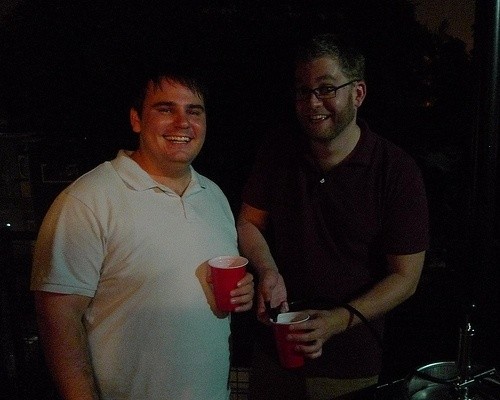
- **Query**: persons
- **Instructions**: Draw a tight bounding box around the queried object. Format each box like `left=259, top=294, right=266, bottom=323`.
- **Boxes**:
left=235, top=32, right=430, bottom=400
left=31, top=61, right=254, bottom=400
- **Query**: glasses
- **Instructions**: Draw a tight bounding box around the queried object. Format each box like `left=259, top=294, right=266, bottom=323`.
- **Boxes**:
left=295, top=79, right=361, bottom=100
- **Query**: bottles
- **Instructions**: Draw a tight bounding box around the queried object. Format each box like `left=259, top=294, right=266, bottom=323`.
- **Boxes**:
left=455, top=317, right=477, bottom=385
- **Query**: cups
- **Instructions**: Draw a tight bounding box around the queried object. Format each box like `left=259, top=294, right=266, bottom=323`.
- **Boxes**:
left=209, top=255, right=248, bottom=312
left=269, top=310, right=310, bottom=369
